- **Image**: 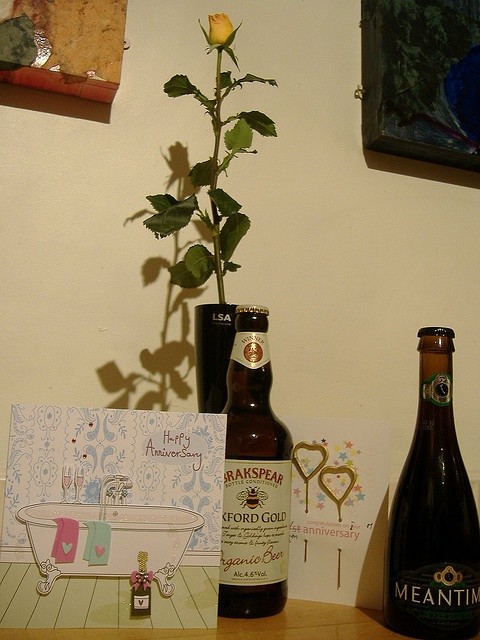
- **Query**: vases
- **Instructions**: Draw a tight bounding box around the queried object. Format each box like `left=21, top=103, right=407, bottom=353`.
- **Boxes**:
left=194, top=301, right=238, bottom=413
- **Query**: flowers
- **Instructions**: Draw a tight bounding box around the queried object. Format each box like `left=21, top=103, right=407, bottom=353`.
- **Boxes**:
left=141, top=12, right=279, bottom=306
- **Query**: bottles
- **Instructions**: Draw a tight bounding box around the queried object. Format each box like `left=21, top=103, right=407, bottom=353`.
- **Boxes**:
left=384, top=327, right=480, bottom=639
left=217, top=304, right=294, bottom=619
